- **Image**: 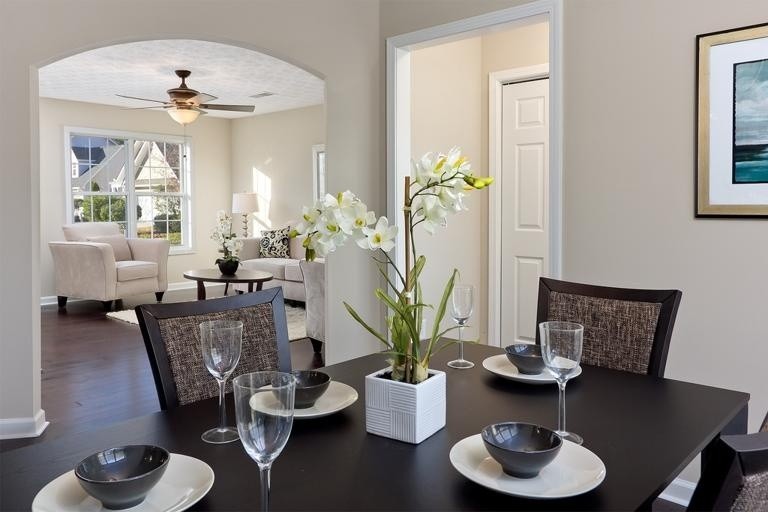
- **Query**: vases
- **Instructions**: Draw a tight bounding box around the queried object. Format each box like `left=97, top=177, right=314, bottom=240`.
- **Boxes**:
left=217, top=260, right=239, bottom=276
left=364, top=364, right=447, bottom=445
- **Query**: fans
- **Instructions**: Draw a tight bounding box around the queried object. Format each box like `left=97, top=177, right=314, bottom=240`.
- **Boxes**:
left=114, top=70, right=255, bottom=115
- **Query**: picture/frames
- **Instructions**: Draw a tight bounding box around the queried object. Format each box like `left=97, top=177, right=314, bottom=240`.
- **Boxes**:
left=693, top=22, right=767, bottom=220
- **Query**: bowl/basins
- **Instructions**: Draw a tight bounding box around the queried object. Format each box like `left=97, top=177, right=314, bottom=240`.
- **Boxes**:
left=478, top=422, right=563, bottom=479
left=73, top=444, right=170, bottom=509
left=505, top=344, right=555, bottom=374
left=268, top=370, right=333, bottom=408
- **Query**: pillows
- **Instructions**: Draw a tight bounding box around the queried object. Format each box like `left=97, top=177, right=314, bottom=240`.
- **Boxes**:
left=259, top=225, right=292, bottom=259
left=87, top=234, right=133, bottom=261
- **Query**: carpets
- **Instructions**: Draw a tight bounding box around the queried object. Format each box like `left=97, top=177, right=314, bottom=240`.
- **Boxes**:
left=105, top=284, right=309, bottom=343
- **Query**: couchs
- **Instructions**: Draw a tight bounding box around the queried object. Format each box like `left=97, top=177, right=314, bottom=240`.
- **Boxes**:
left=232, top=234, right=308, bottom=302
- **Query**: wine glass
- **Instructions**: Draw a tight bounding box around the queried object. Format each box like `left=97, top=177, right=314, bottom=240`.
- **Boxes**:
left=538, top=320, right=584, bottom=450
left=199, top=320, right=243, bottom=443
left=230, top=371, right=296, bottom=512
left=446, top=284, right=476, bottom=370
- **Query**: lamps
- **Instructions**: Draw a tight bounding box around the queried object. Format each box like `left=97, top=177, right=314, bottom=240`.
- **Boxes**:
left=232, top=191, right=259, bottom=237
left=167, top=85, right=201, bottom=125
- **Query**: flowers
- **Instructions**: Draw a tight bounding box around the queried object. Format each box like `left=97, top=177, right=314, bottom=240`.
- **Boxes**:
left=288, top=145, right=495, bottom=383
left=209, top=209, right=243, bottom=266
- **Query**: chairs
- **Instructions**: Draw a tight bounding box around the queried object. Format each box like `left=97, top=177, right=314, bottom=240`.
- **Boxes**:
left=534, top=276, right=682, bottom=512
left=135, top=286, right=292, bottom=408
left=299, top=256, right=326, bottom=350
left=685, top=413, right=768, bottom=512
left=47, top=221, right=171, bottom=312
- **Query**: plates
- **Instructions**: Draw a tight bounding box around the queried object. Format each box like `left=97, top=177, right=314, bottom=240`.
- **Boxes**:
left=446, top=433, right=607, bottom=499
left=245, top=380, right=359, bottom=419
left=481, top=352, right=583, bottom=390
left=32, top=453, right=216, bottom=512
